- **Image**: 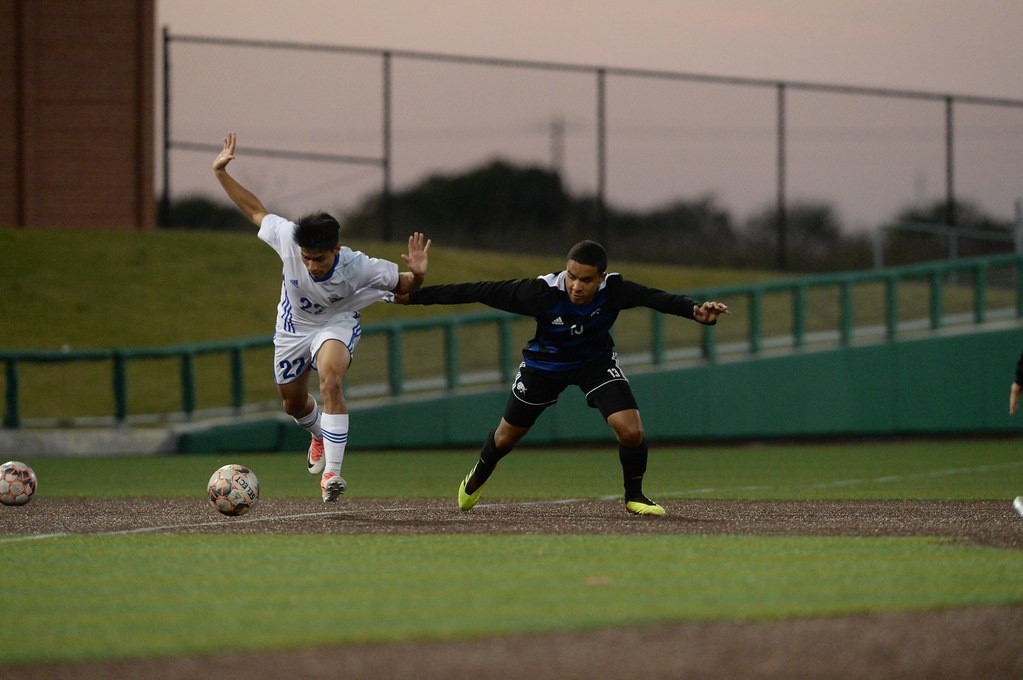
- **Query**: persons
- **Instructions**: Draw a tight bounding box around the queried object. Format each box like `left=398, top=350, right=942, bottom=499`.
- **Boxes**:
left=212, top=132, right=431, bottom=501
left=1009, top=355, right=1023, bottom=516
left=392, top=240, right=731, bottom=515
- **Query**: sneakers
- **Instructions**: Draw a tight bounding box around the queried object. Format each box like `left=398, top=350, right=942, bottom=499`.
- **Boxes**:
left=458, top=462, right=497, bottom=511
left=625, top=493, right=666, bottom=515
left=307, top=431, right=326, bottom=474
left=321, top=470, right=346, bottom=503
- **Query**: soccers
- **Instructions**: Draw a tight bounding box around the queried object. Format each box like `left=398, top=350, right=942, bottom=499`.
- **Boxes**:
left=206, top=464, right=259, bottom=517
left=0, top=461, right=37, bottom=507
